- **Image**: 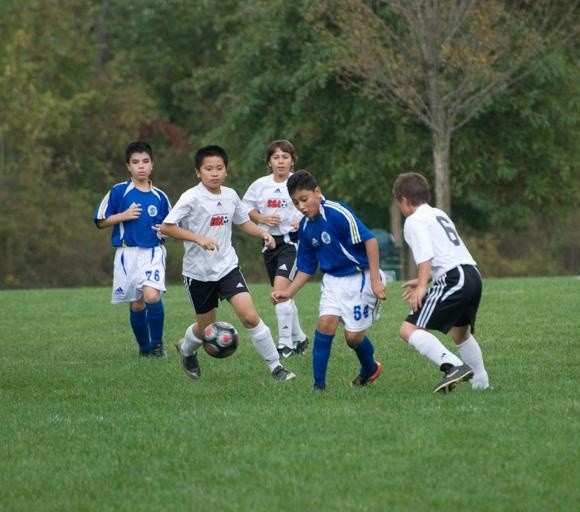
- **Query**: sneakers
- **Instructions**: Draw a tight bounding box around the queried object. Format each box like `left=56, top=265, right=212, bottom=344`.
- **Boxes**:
left=140, top=344, right=166, bottom=358
left=271, top=336, right=383, bottom=391
left=176, top=339, right=202, bottom=378
left=432, top=363, right=475, bottom=393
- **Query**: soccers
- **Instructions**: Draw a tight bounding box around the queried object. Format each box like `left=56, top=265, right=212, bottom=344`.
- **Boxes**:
left=202, top=322, right=239, bottom=357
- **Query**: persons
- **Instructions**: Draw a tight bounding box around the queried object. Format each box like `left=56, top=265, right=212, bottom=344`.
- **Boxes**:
left=160, top=144, right=296, bottom=381
left=242, top=141, right=324, bottom=359
left=270, top=173, right=386, bottom=390
left=392, top=172, right=490, bottom=393
left=94, top=140, right=178, bottom=358
